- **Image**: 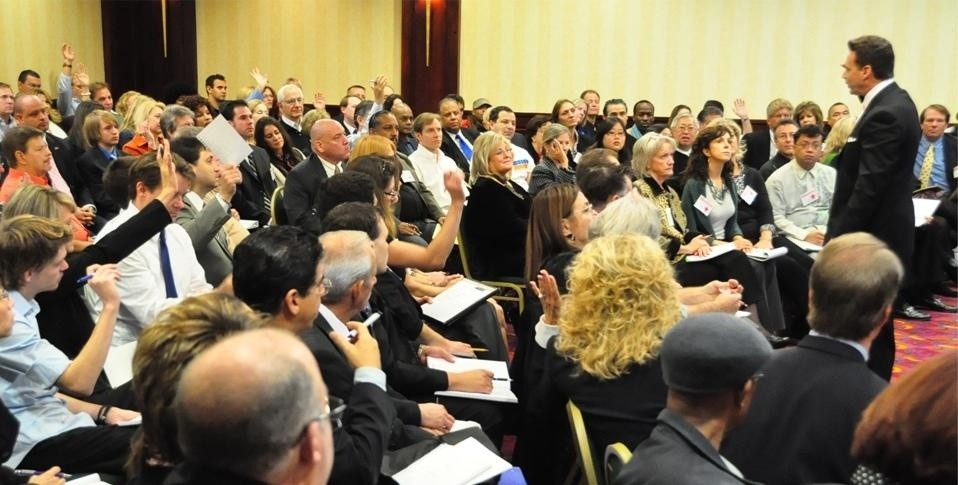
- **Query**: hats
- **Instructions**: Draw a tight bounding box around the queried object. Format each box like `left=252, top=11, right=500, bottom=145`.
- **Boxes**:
left=472, top=98, right=492, bottom=109
left=660, top=312, right=774, bottom=395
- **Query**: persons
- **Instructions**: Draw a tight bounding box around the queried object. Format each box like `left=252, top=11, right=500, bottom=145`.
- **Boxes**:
left=383, top=85, right=394, bottom=105
left=447, top=94, right=465, bottom=118
left=0, top=213, right=142, bottom=476
left=302, top=107, right=332, bottom=138
left=15, top=69, right=41, bottom=99
left=552, top=98, right=578, bottom=129
left=347, top=84, right=367, bottom=102
left=179, top=95, right=213, bottom=128
left=56, top=43, right=92, bottom=133
left=589, top=194, right=744, bottom=317
left=384, top=94, right=405, bottom=111
left=204, top=74, right=227, bottom=119
left=528, top=124, right=576, bottom=192
left=764, top=125, right=838, bottom=259
left=602, top=98, right=628, bottom=127
left=322, top=201, right=508, bottom=452
left=577, top=147, right=620, bottom=169
left=285, top=77, right=304, bottom=89
left=0, top=82, right=19, bottom=141
left=247, top=98, right=270, bottom=123
left=470, top=97, right=492, bottom=132
left=102, top=155, right=141, bottom=213
left=528, top=233, right=693, bottom=484
left=823, top=34, right=921, bottom=381
left=89, top=81, right=116, bottom=114
left=63, top=100, right=105, bottom=160
left=78, top=150, right=233, bottom=346
left=367, top=109, right=466, bottom=276
left=719, top=231, right=904, bottom=484
left=461, top=131, right=534, bottom=314
left=932, top=215, right=957, bottom=297
left=159, top=104, right=195, bottom=141
left=647, top=123, right=672, bottom=138
left=794, top=101, right=823, bottom=129
left=489, top=105, right=516, bottom=140
left=679, top=125, right=752, bottom=255
left=760, top=119, right=801, bottom=182
left=122, top=100, right=166, bottom=156
left=526, top=117, right=547, bottom=164
left=408, top=111, right=469, bottom=273
left=115, top=91, right=139, bottom=116
left=849, top=348, right=958, bottom=485
left=571, top=98, right=594, bottom=152
left=317, top=171, right=508, bottom=364
left=0, top=139, right=179, bottom=412
left=174, top=137, right=243, bottom=288
left=117, top=96, right=157, bottom=151
left=283, top=120, right=351, bottom=224
left=700, top=99, right=724, bottom=112
left=221, top=99, right=256, bottom=139
left=255, top=116, right=307, bottom=198
left=618, top=100, right=656, bottom=164
left=819, top=116, right=857, bottom=165
left=696, top=99, right=754, bottom=136
left=164, top=326, right=345, bottom=485
left=586, top=116, right=631, bottom=165
left=391, top=103, right=419, bottom=156
left=176, top=127, right=204, bottom=139
left=0, top=125, right=96, bottom=252
left=893, top=214, right=958, bottom=320
left=353, top=73, right=388, bottom=135
left=348, top=134, right=396, bottom=166
left=232, top=224, right=456, bottom=485
left=0, top=274, right=127, bottom=485
left=316, top=229, right=456, bottom=431
left=580, top=89, right=602, bottom=134
left=766, top=98, right=794, bottom=129
left=247, top=67, right=280, bottom=120
left=509, top=182, right=600, bottom=323
left=708, top=118, right=786, bottom=333
left=438, top=98, right=479, bottom=170
left=133, top=292, right=269, bottom=485
left=670, top=114, right=700, bottom=177
left=32, top=88, right=68, bottom=139
left=71, top=109, right=128, bottom=219
left=273, top=85, right=326, bottom=158
left=741, top=117, right=749, bottom=122
left=614, top=312, right=773, bottom=485
left=336, top=94, right=362, bottom=136
left=345, top=154, right=468, bottom=282
left=911, top=103, right=958, bottom=215
left=0, top=92, right=107, bottom=235
left=632, top=130, right=792, bottom=349
left=482, top=106, right=527, bottom=151
left=826, top=101, right=852, bottom=127
left=667, top=104, right=692, bottom=129
left=577, top=160, right=633, bottom=214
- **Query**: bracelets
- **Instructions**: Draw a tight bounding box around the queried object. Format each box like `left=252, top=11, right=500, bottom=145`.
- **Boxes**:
left=559, top=159, right=568, bottom=163
left=97, top=406, right=105, bottom=424
left=222, top=197, right=232, bottom=207
left=417, top=344, right=424, bottom=357
left=63, top=62, right=72, bottom=68
left=100, top=406, right=111, bottom=424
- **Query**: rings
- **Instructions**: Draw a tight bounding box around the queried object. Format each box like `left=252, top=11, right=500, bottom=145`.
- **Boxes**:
left=538, top=293, right=545, bottom=298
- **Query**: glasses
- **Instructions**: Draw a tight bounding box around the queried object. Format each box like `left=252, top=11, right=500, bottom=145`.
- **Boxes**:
left=310, top=276, right=333, bottom=290
left=384, top=189, right=397, bottom=196
left=289, top=394, right=346, bottom=449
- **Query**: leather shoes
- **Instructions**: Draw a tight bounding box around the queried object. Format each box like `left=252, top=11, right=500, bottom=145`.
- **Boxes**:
left=916, top=298, right=955, bottom=313
left=893, top=306, right=932, bottom=321
left=933, top=286, right=958, bottom=298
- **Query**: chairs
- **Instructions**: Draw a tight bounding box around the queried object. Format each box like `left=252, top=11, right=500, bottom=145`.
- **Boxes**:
left=602, top=441, right=634, bottom=482
left=261, top=164, right=287, bottom=224
left=442, top=217, right=528, bottom=314
left=561, top=402, right=600, bottom=485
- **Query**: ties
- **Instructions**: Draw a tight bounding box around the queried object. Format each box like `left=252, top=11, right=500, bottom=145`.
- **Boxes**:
left=334, top=166, right=340, bottom=175
left=242, top=151, right=278, bottom=207
left=918, top=143, right=936, bottom=189
left=158, top=227, right=178, bottom=299
left=456, top=134, right=473, bottom=161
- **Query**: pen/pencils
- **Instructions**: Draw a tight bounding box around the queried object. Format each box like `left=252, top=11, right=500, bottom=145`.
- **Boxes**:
left=812, top=224, right=818, bottom=229
left=14, top=468, right=75, bottom=479
left=718, top=288, right=748, bottom=307
left=703, top=233, right=716, bottom=238
left=347, top=310, right=383, bottom=343
left=493, top=376, right=516, bottom=383
left=75, top=274, right=95, bottom=284
